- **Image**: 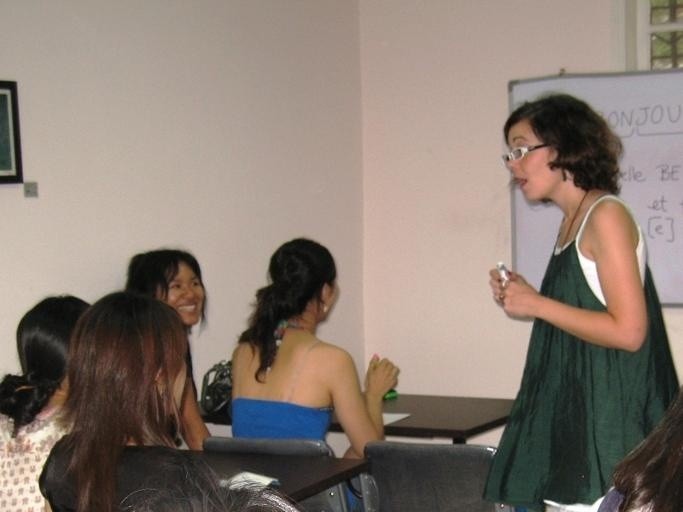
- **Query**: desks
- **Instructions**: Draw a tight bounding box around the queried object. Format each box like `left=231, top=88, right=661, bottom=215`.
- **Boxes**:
left=87, top=393, right=516, bottom=512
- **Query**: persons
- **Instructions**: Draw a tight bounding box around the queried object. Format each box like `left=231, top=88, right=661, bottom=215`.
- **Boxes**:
left=114, top=462, right=303, bottom=511
left=593, top=385, right=683, bottom=511
left=116, top=247, right=214, bottom=455
left=486, top=91, right=682, bottom=510
left=32, top=290, right=208, bottom=512
left=229, top=236, right=401, bottom=511
left=0, top=292, right=95, bottom=511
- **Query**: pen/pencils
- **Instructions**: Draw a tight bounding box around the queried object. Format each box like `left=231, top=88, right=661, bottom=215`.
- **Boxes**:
left=498, top=263, right=506, bottom=285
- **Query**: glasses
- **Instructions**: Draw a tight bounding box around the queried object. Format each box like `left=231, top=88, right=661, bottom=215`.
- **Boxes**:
left=500, top=141, right=555, bottom=167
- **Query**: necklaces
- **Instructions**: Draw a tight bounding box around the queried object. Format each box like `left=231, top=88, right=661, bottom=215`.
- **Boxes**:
left=560, top=190, right=589, bottom=254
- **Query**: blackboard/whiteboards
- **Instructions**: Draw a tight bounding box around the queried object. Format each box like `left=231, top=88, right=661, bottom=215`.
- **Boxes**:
left=510, top=69, right=683, bottom=307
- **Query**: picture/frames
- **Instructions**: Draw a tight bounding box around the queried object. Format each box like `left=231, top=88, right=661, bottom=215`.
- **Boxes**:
left=0, top=80, right=23, bottom=184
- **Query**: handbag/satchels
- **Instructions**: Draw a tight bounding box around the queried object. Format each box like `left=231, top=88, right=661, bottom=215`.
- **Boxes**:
left=199, top=357, right=235, bottom=425
